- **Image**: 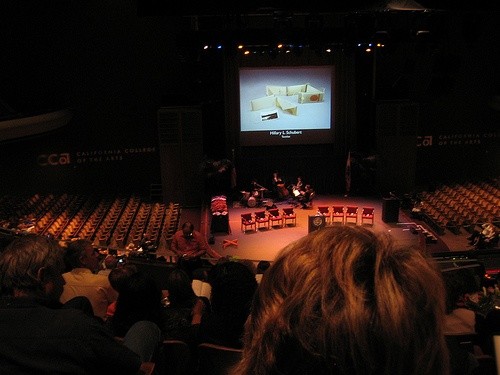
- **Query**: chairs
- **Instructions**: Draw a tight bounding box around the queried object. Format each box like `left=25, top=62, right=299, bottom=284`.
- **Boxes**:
left=282, top=208, right=297, bottom=227
left=268, top=209, right=283, bottom=228
left=116, top=299, right=243, bottom=375
left=345, top=207, right=359, bottom=223
left=240, top=213, right=256, bottom=234
left=254, top=211, right=270, bottom=230
left=361, top=208, right=375, bottom=225
left=332, top=206, right=345, bottom=222
left=317, top=206, right=331, bottom=222
left=403, top=174, right=500, bottom=235
left=0, top=194, right=181, bottom=250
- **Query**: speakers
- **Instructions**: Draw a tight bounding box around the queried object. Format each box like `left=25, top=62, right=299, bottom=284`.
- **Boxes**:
left=382, top=198, right=400, bottom=223
left=211, top=212, right=229, bottom=234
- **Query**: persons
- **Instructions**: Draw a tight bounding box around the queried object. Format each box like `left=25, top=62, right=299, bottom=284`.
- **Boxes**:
left=252, top=173, right=312, bottom=209
left=465, top=218, right=496, bottom=248
left=171, top=223, right=207, bottom=262
left=0, top=232, right=500, bottom=375
